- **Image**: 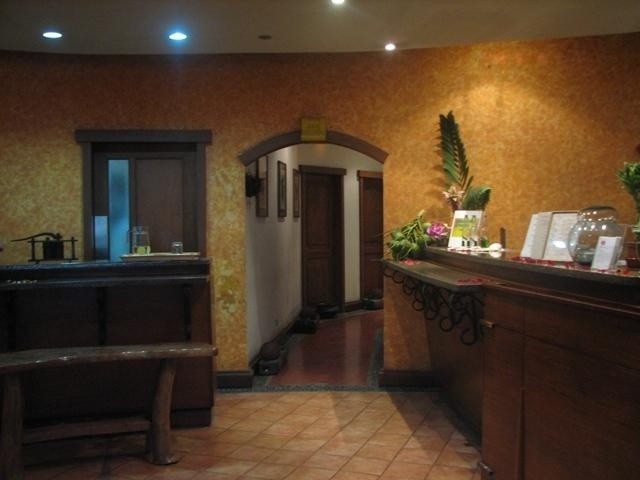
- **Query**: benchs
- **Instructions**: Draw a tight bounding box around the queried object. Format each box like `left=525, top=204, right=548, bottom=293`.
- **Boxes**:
left=1, top=340, right=220, bottom=479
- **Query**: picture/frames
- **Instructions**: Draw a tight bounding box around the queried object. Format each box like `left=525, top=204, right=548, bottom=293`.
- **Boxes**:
left=255, top=156, right=301, bottom=219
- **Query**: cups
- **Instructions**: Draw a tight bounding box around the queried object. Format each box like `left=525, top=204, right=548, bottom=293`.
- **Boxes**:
left=131, top=225, right=152, bottom=255
left=172, top=242, right=184, bottom=254
